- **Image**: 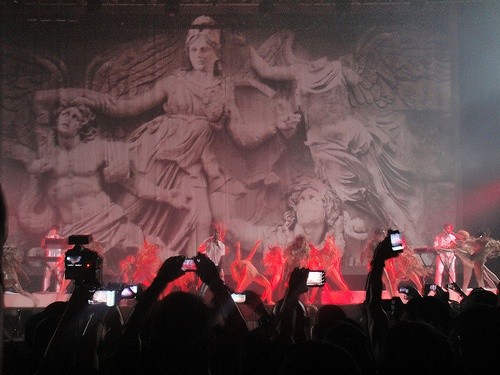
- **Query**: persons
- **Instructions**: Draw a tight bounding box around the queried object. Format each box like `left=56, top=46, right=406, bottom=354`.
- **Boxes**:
left=26, top=87, right=144, bottom=264
left=200, top=142, right=347, bottom=266
left=227, top=30, right=433, bottom=266
left=69, top=15, right=301, bottom=260
left=0, top=222, right=500, bottom=375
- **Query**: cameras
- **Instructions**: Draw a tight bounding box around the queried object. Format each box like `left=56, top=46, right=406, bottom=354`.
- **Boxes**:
left=429, top=284, right=437, bottom=291
left=231, top=292, right=247, bottom=303
left=446, top=284, right=453, bottom=288
left=398, top=287, right=410, bottom=294
left=181, top=258, right=200, bottom=272
left=389, top=230, right=404, bottom=254
left=305, top=270, right=325, bottom=287
left=88, top=281, right=126, bottom=308
left=120, top=285, right=139, bottom=298
